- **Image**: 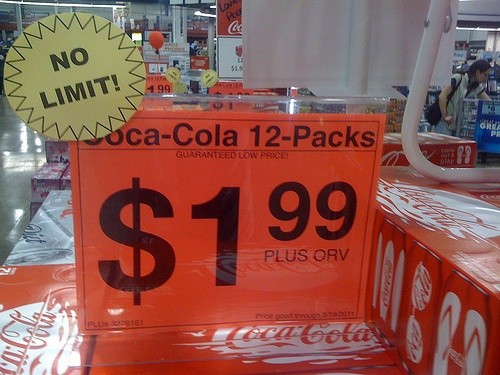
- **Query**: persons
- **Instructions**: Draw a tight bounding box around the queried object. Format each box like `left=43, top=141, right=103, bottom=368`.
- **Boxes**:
left=430, top=60, right=492, bottom=137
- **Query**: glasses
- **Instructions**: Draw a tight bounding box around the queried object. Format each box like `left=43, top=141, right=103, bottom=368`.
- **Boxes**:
left=482, top=72, right=490, bottom=78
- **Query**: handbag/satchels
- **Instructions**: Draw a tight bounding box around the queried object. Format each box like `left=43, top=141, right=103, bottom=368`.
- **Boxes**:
left=425, top=99, right=443, bottom=125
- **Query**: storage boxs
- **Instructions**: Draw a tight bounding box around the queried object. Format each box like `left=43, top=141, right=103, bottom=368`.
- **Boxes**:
left=30, top=139, right=72, bottom=221
left=382, top=130, right=477, bottom=170
left=359, top=166, right=500, bottom=375
